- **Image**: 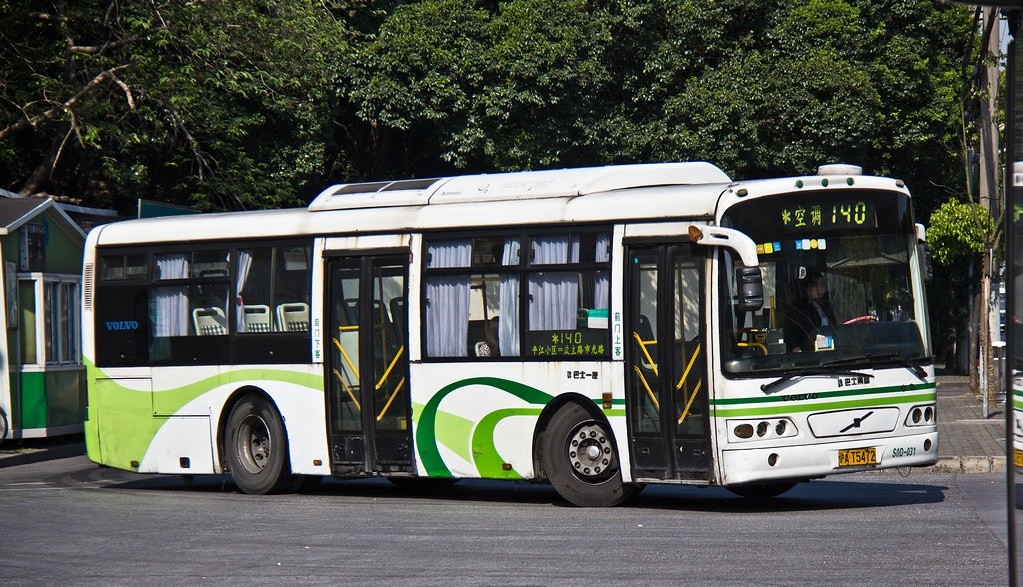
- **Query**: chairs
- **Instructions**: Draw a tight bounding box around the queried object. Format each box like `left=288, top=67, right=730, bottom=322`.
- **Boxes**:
left=337, top=296, right=406, bottom=362
left=639, top=314, right=658, bottom=374
left=193, top=307, right=226, bottom=336
left=277, top=302, right=309, bottom=332
left=242, top=305, right=277, bottom=331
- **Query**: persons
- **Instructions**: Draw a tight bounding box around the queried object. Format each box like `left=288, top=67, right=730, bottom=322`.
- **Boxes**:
left=783, top=273, right=836, bottom=352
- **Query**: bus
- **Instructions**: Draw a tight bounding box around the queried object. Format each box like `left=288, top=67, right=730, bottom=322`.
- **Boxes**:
left=77, top=161, right=941, bottom=509
